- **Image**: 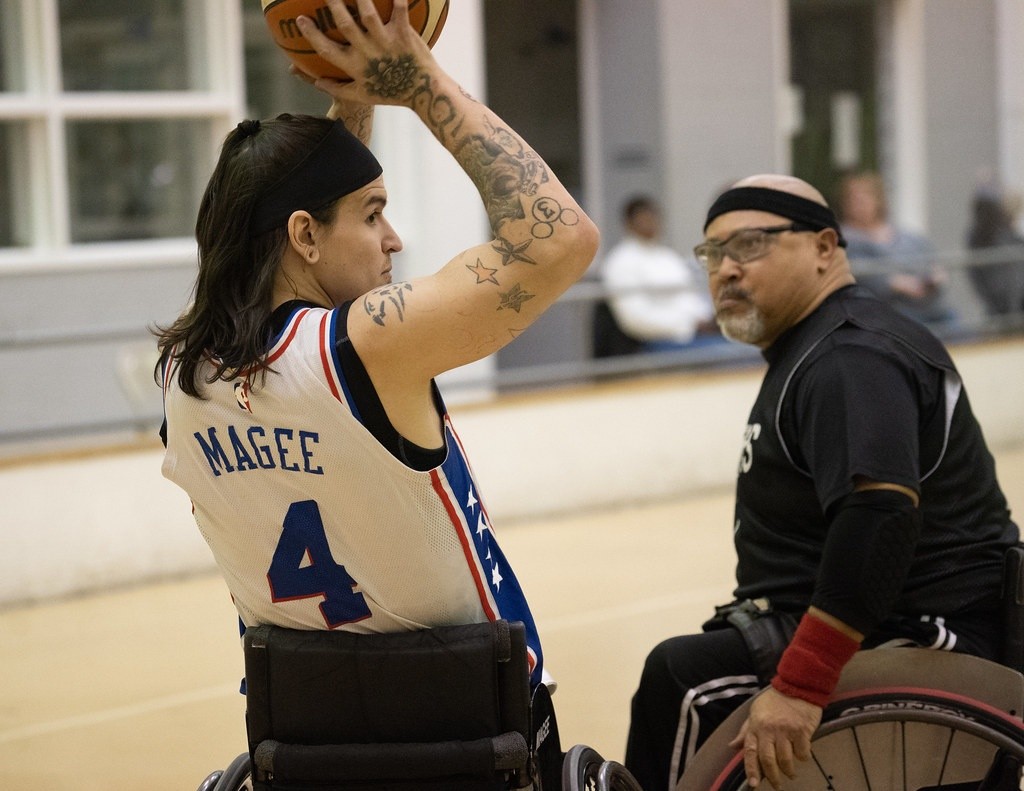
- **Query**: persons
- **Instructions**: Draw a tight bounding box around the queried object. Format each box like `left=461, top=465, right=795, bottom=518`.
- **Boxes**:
left=619, top=171, right=1024, bottom=791
left=963, top=186, right=1024, bottom=316
left=603, top=191, right=734, bottom=348
left=159, top=0, right=604, bottom=789
left=833, top=171, right=966, bottom=323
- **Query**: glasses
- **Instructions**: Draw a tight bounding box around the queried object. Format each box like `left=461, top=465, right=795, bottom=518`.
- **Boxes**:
left=693, top=222, right=848, bottom=272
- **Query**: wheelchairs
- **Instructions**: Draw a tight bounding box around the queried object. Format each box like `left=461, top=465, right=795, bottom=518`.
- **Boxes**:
left=668, top=542, right=1024, bottom=791
left=197, top=617, right=642, bottom=791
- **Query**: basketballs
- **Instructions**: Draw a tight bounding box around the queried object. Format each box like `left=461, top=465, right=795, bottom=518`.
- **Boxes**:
left=258, top=1, right=450, bottom=82
